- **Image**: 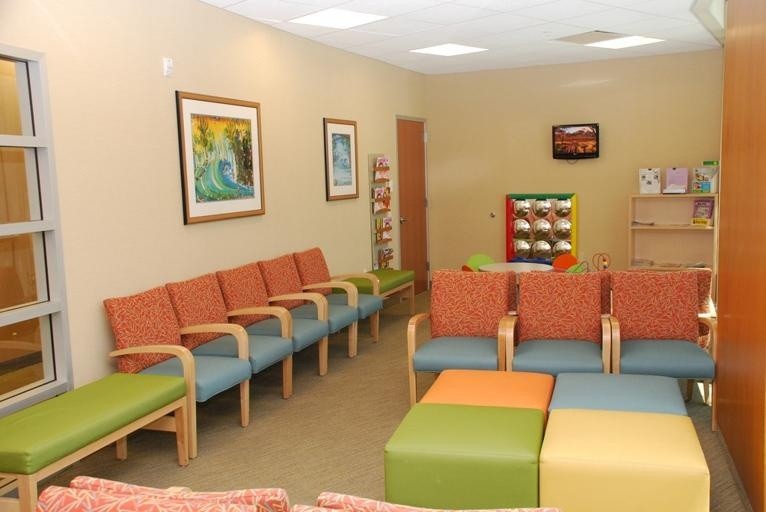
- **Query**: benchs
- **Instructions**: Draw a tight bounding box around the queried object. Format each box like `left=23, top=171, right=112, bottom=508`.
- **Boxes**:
left=0, top=371, right=189, bottom=511
left=332, top=268, right=416, bottom=344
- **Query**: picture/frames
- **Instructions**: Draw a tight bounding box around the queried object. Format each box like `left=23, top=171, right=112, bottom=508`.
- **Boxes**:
left=173, top=86, right=267, bottom=226
left=322, top=115, right=360, bottom=201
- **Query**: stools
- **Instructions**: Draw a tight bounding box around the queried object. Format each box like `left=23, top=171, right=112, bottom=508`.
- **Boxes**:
left=538, top=409, right=712, bottom=512
left=380, top=402, right=545, bottom=508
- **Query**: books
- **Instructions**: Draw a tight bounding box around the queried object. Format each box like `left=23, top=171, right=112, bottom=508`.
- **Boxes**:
left=371, top=157, right=393, bottom=269
left=636, top=161, right=715, bottom=227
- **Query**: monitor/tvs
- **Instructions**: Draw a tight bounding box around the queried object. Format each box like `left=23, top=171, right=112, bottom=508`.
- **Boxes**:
left=552, top=122, right=599, bottom=159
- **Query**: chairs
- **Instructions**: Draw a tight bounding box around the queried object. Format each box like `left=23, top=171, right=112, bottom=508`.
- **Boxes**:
left=506, top=272, right=611, bottom=374
left=601, top=272, right=611, bottom=315
left=105, top=286, right=250, bottom=459
left=698, top=270, right=712, bottom=315
left=259, top=255, right=358, bottom=376
left=408, top=271, right=509, bottom=405
left=165, top=274, right=294, bottom=427
left=508, top=271, right=519, bottom=316
left=294, top=248, right=383, bottom=358
left=610, top=270, right=718, bottom=430
left=217, top=264, right=328, bottom=400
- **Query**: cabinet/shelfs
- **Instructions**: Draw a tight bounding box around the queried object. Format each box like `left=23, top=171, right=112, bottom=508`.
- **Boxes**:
left=373, top=165, right=393, bottom=267
left=629, top=195, right=719, bottom=303
